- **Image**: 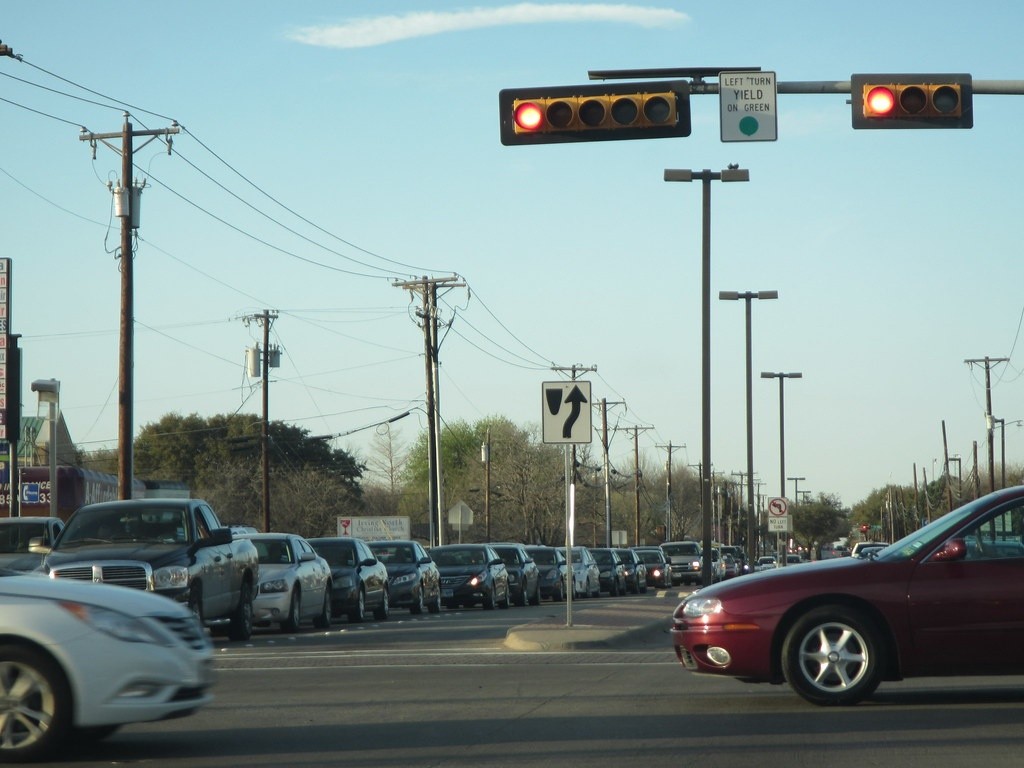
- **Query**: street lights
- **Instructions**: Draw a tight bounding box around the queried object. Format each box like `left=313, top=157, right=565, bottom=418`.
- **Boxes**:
left=797, top=491, right=811, bottom=502
left=949, top=457, right=962, bottom=507
left=719, top=290, right=779, bottom=574
left=787, top=478, right=805, bottom=508
left=759, top=372, right=802, bottom=566
left=664, top=165, right=751, bottom=581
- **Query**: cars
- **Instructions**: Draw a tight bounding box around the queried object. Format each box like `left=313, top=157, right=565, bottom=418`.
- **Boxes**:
left=0, top=565, right=215, bottom=761
left=242, top=533, right=332, bottom=632
left=851, top=541, right=889, bottom=556
left=489, top=544, right=542, bottom=607
left=523, top=547, right=577, bottom=601
left=426, top=543, right=512, bottom=610
left=667, top=485, right=1024, bottom=707
left=554, top=543, right=775, bottom=598
left=364, top=540, right=442, bottom=615
left=305, top=537, right=391, bottom=625
left=0, top=516, right=65, bottom=575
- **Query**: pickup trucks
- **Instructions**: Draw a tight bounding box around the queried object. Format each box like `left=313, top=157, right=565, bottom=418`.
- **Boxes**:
left=35, top=496, right=259, bottom=642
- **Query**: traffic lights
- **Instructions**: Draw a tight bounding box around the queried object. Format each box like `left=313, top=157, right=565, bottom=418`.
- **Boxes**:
left=850, top=73, right=973, bottom=129
left=498, top=80, right=692, bottom=145
